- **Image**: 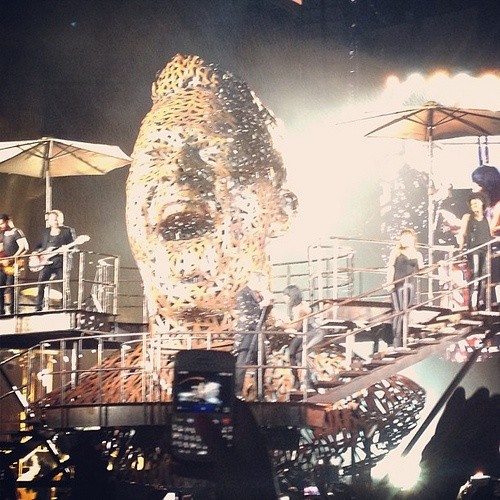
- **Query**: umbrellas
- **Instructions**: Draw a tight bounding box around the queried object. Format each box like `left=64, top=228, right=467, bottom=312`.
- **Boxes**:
left=0, top=134, right=133, bottom=313
left=342, top=101, right=500, bottom=306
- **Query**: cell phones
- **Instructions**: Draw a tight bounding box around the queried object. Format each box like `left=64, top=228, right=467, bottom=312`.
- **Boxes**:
left=170, top=349, right=236, bottom=461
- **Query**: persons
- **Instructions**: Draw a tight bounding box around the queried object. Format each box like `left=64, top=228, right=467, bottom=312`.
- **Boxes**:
left=28, top=210, right=79, bottom=312
left=0, top=387, right=500, bottom=500
left=457, top=165, right=499, bottom=245
left=384, top=227, right=435, bottom=352
left=229, top=273, right=278, bottom=404
left=18, top=51, right=429, bottom=483
left=456, top=192, right=500, bottom=318
left=275, top=285, right=327, bottom=394
left=0, top=212, right=30, bottom=315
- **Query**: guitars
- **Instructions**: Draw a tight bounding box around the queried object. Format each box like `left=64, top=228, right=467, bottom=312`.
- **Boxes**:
left=29, top=235, right=90, bottom=272
left=0, top=252, right=24, bottom=275
